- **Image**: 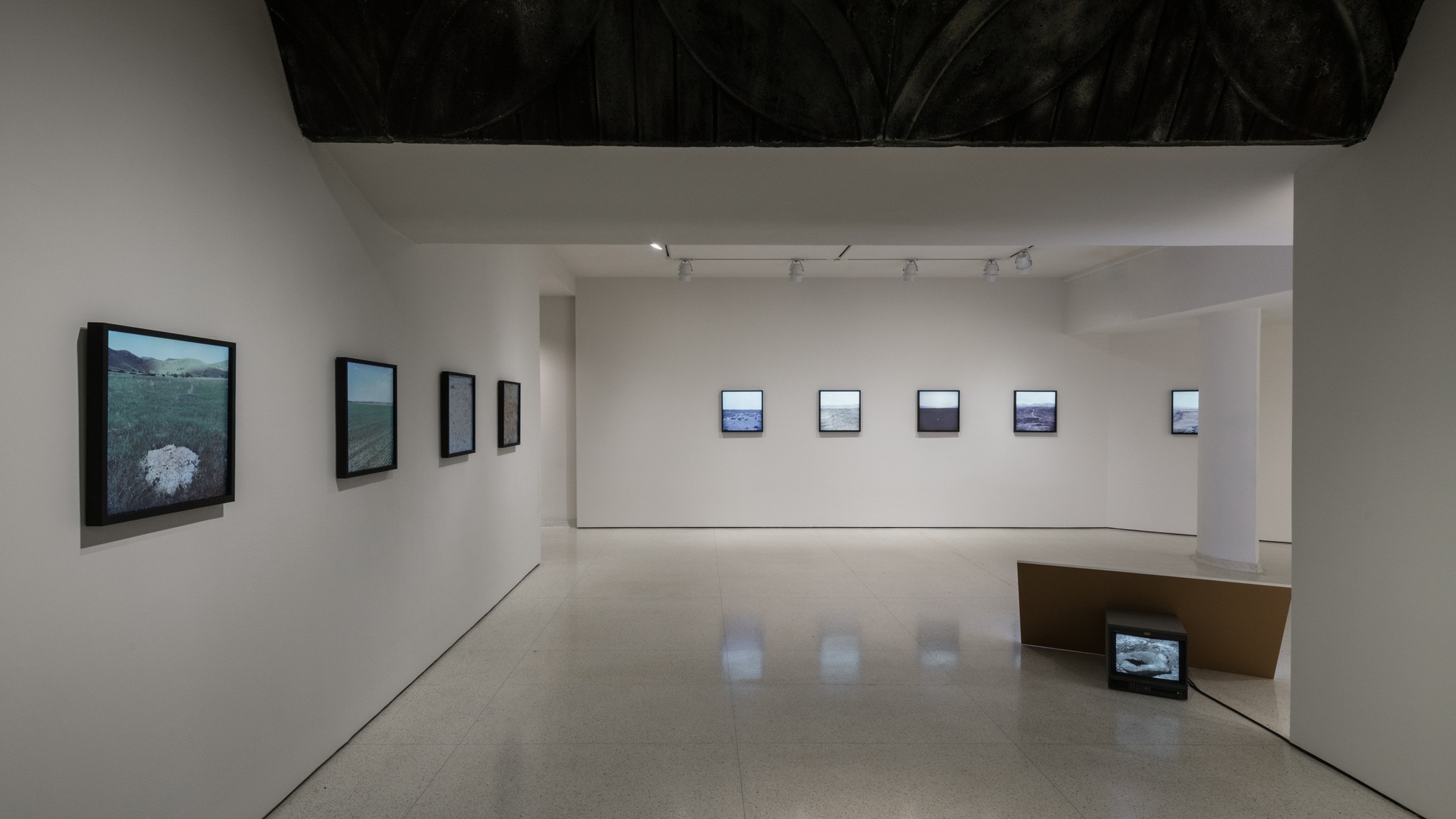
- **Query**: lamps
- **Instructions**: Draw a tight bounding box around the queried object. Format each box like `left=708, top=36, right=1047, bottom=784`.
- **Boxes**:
left=678, top=250, right=1035, bottom=282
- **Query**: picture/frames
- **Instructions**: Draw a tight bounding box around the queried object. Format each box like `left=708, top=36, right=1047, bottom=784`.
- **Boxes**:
left=918, top=390, right=961, bottom=433
left=497, top=377, right=523, bottom=447
left=84, top=319, right=237, bottom=527
left=441, top=370, right=477, bottom=459
left=1170, top=389, right=1199, bottom=434
left=335, top=356, right=399, bottom=480
left=1014, top=390, right=1059, bottom=434
left=818, top=390, right=861, bottom=432
left=720, top=390, right=765, bottom=432
